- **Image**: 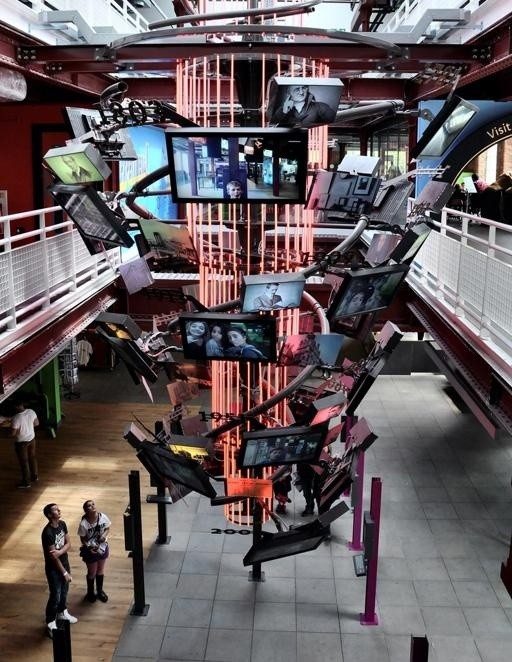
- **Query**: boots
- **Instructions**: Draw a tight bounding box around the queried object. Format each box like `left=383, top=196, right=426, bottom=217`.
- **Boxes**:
left=95, top=574, right=108, bottom=601
left=86, top=574, right=96, bottom=603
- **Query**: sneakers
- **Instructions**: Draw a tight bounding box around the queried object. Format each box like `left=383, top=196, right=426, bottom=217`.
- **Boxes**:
left=277, top=505, right=286, bottom=515
left=47, top=621, right=58, bottom=638
left=302, top=508, right=312, bottom=516
left=17, top=483, right=30, bottom=489
left=57, top=609, right=78, bottom=624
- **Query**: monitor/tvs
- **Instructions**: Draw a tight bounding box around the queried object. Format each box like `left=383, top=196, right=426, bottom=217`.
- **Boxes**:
left=63, top=106, right=137, bottom=161
left=138, top=220, right=201, bottom=266
left=388, top=224, right=431, bottom=266
left=325, top=264, right=411, bottom=324
left=304, top=170, right=382, bottom=217
left=265, top=76, right=344, bottom=126
left=410, top=96, right=480, bottom=159
left=178, top=312, right=278, bottom=364
left=50, top=184, right=134, bottom=249
left=414, top=181, right=455, bottom=214
left=95, top=312, right=143, bottom=342
left=336, top=153, right=382, bottom=176
left=116, top=257, right=156, bottom=296
left=278, top=321, right=404, bottom=416
left=77, top=207, right=130, bottom=256
left=42, top=142, right=112, bottom=185
left=236, top=273, right=304, bottom=312
left=121, top=389, right=432, bottom=567
left=165, top=127, right=309, bottom=205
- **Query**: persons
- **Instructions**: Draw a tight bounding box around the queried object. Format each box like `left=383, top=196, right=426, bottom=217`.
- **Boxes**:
left=206, top=324, right=228, bottom=356
left=41, top=503, right=79, bottom=636
left=337, top=284, right=374, bottom=313
left=269, top=438, right=290, bottom=456
left=460, top=182, right=469, bottom=191
left=78, top=500, right=112, bottom=602
left=62, top=155, right=92, bottom=183
left=471, top=174, right=487, bottom=194
left=271, top=85, right=335, bottom=124
left=254, top=282, right=298, bottom=310
left=105, top=322, right=132, bottom=341
left=226, top=181, right=242, bottom=199
left=255, top=447, right=286, bottom=464
left=298, top=462, right=328, bottom=515
left=186, top=321, right=209, bottom=356
left=271, top=300, right=296, bottom=308
left=11, top=398, right=41, bottom=487
left=223, top=326, right=268, bottom=358
left=453, top=184, right=467, bottom=203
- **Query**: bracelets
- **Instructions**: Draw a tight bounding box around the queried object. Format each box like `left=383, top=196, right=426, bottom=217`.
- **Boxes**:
left=63, top=571, right=69, bottom=576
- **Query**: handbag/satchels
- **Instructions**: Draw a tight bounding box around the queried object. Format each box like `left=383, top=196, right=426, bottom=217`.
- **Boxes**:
left=80, top=546, right=89, bottom=556
